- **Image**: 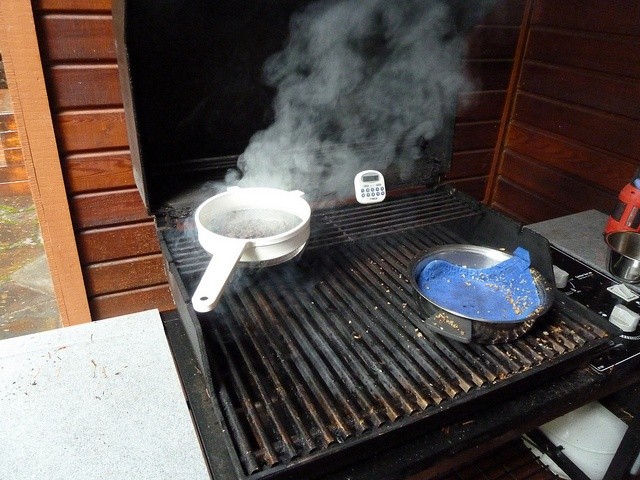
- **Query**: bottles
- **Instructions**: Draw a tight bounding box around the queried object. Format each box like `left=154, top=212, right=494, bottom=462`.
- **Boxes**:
left=603, top=165, right=640, bottom=236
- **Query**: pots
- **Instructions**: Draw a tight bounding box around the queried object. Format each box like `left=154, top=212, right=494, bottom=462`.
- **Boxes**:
left=408, top=244, right=557, bottom=345
left=192, top=187, right=312, bottom=312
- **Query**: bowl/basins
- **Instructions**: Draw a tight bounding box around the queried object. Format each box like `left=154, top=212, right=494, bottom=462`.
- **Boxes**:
left=605, top=230, right=640, bottom=283
